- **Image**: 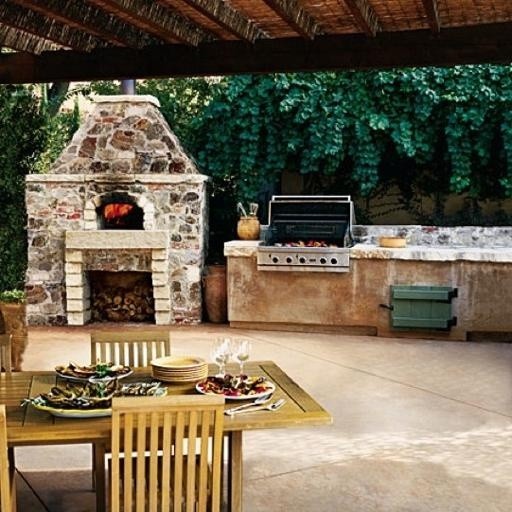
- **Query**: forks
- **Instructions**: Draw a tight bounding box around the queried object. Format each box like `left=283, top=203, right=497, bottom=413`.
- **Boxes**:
left=225, top=398, right=288, bottom=416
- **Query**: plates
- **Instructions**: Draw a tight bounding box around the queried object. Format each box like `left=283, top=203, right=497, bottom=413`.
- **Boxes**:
left=194, top=373, right=277, bottom=401
left=29, top=383, right=170, bottom=419
left=149, top=354, right=210, bottom=385
left=54, top=360, right=135, bottom=382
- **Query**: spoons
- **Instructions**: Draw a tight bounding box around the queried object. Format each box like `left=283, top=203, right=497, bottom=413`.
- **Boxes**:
left=222, top=392, right=274, bottom=414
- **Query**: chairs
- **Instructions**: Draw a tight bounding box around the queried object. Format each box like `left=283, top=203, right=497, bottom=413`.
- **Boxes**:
left=88, top=328, right=174, bottom=512
left=1, top=332, right=17, bottom=511
left=105, top=393, right=226, bottom=511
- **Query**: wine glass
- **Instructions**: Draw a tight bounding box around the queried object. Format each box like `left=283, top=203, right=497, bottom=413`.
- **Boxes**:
left=233, top=338, right=253, bottom=377
left=210, top=335, right=234, bottom=379
